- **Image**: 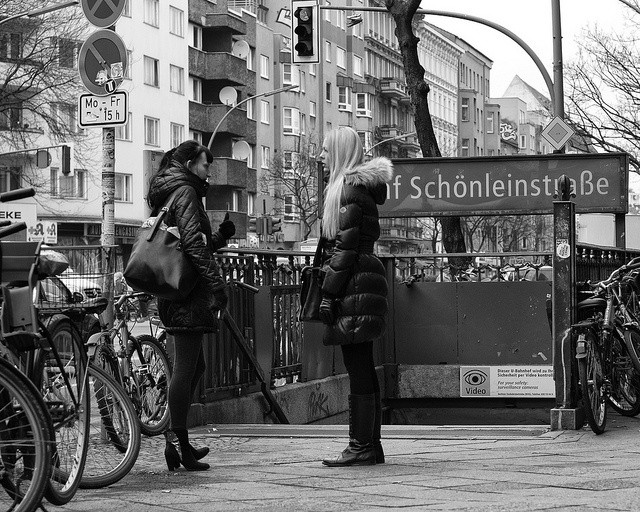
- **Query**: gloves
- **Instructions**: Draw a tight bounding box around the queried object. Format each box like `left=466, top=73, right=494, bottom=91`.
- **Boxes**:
left=211, top=288, right=228, bottom=310
left=219, top=211, right=235, bottom=239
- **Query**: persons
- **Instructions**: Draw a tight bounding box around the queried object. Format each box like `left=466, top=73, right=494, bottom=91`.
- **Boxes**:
left=147, top=139, right=236, bottom=472
left=318, top=127, right=394, bottom=466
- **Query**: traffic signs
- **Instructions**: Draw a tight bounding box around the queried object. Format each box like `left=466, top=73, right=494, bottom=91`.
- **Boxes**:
left=78, top=91, right=128, bottom=129
left=78, top=29, right=128, bottom=97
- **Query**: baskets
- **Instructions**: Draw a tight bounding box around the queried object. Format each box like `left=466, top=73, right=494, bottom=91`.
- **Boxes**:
left=32, top=244, right=118, bottom=314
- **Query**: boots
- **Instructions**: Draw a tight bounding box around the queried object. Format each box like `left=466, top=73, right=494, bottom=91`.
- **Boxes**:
left=322, top=393, right=385, bottom=467
left=175, top=443, right=209, bottom=469
left=162, top=428, right=210, bottom=471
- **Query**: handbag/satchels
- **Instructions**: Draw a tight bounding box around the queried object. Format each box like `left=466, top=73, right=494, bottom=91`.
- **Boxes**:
left=123, top=186, right=196, bottom=301
left=299, top=266, right=326, bottom=324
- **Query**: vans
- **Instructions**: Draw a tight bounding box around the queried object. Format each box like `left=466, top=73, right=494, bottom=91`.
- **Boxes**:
left=52, top=262, right=103, bottom=303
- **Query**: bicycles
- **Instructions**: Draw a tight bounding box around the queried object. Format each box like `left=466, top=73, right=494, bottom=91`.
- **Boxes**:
left=392, top=256, right=551, bottom=288
left=137, top=319, right=167, bottom=424
left=0, top=217, right=61, bottom=512
left=218, top=263, right=301, bottom=394
left=570, top=254, right=640, bottom=436
left=0, top=273, right=141, bottom=506
left=86, top=291, right=177, bottom=451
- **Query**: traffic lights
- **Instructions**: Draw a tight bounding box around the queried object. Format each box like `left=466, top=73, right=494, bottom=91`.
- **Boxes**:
left=263, top=215, right=282, bottom=237
left=290, top=0, right=321, bottom=65
left=248, top=215, right=262, bottom=237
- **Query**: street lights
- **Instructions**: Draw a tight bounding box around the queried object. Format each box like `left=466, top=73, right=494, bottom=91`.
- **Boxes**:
left=205, top=84, right=299, bottom=153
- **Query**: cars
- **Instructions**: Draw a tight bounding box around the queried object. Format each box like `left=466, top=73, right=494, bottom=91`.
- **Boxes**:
left=31, top=270, right=101, bottom=339
